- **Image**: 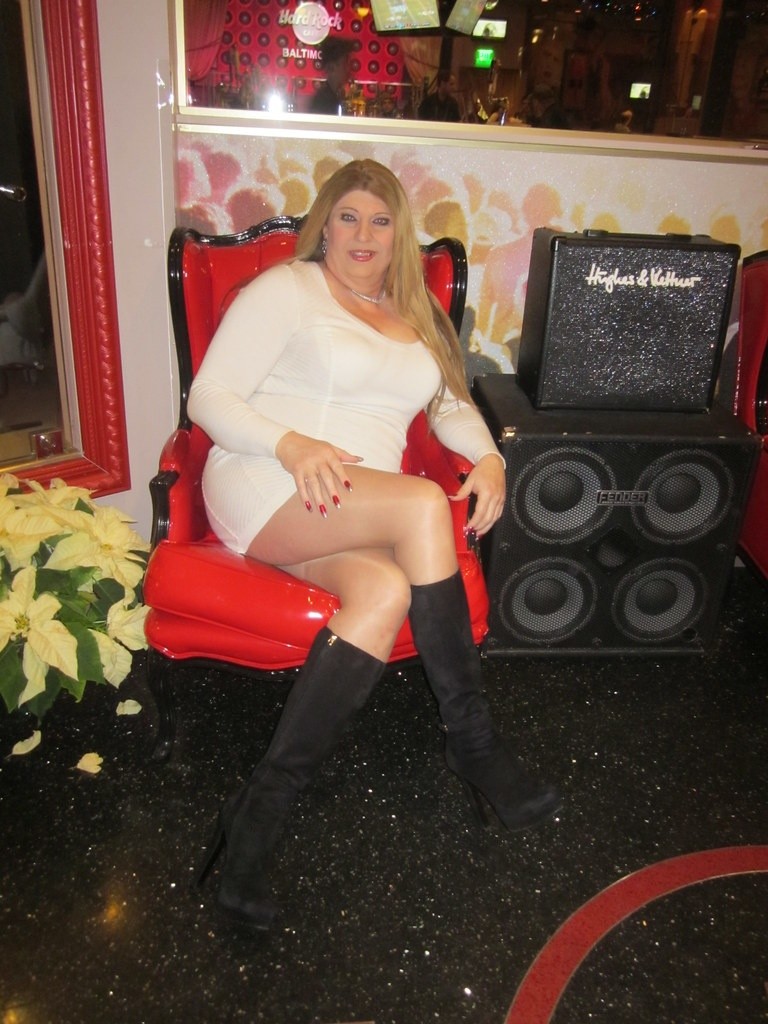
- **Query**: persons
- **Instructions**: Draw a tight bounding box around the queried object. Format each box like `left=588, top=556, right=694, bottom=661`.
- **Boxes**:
left=417, top=71, right=461, bottom=122
left=183, top=158, right=565, bottom=919
left=379, top=97, right=401, bottom=118
left=310, top=39, right=356, bottom=116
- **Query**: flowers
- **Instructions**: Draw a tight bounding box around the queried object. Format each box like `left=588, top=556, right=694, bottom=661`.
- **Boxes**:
left=0, top=463, right=151, bottom=772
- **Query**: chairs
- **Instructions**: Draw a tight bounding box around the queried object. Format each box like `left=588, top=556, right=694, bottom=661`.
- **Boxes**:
left=144, top=220, right=476, bottom=743
left=732, top=251, right=768, bottom=579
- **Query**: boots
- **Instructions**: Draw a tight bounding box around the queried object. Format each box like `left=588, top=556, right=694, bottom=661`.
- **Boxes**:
left=409, top=570, right=563, bottom=834
left=194, top=627, right=386, bottom=928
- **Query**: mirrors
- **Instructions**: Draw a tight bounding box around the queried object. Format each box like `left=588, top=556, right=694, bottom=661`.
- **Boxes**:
left=0, top=0, right=131, bottom=502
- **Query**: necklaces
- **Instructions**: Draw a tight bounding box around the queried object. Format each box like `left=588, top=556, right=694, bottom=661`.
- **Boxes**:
left=352, top=291, right=387, bottom=304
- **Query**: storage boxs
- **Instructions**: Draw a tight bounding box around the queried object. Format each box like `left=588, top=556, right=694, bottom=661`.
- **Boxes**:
left=517, top=227, right=741, bottom=407
